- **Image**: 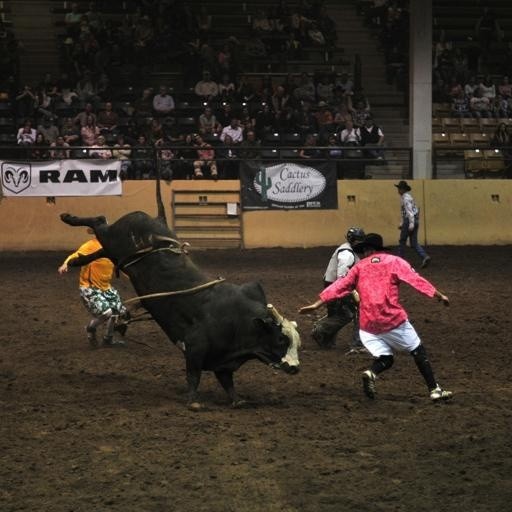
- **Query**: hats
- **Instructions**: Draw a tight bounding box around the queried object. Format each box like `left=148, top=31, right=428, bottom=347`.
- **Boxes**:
left=394, top=181, right=411, bottom=192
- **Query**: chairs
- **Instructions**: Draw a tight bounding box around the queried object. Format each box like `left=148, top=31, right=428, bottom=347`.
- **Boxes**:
left=433, top=102, right=511, bottom=178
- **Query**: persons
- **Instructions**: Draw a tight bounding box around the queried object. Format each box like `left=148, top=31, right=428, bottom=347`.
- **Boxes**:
left=2, top=1, right=388, bottom=178
left=309, top=227, right=363, bottom=353
left=353, top=1, right=512, bottom=179
left=394, top=181, right=431, bottom=269
left=56, top=238, right=128, bottom=345
left=298, top=233, right=453, bottom=401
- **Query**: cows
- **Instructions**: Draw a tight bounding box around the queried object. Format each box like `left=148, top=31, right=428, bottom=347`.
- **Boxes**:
left=59, top=209, right=303, bottom=413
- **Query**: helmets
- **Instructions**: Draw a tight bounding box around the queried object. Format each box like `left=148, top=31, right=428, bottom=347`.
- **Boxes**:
left=346, top=227, right=366, bottom=245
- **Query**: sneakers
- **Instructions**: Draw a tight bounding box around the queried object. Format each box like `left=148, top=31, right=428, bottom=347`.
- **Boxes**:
left=361, top=370, right=377, bottom=399
left=311, top=323, right=335, bottom=352
left=428, top=383, right=454, bottom=402
left=100, top=335, right=127, bottom=348
left=420, top=256, right=431, bottom=269
left=85, top=326, right=97, bottom=345
